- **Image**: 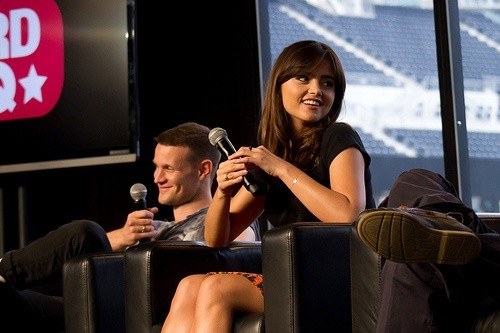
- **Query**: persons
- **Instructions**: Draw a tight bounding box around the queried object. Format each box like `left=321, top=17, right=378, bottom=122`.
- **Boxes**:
left=162, top=41, right=375, bottom=333
left=0, top=122, right=260, bottom=333
left=357, top=168, right=500, bottom=333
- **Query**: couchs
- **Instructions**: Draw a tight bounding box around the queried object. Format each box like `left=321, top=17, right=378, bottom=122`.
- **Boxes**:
left=62, top=216, right=500, bottom=333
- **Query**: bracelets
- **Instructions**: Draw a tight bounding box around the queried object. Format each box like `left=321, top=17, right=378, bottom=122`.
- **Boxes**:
left=289, top=170, right=303, bottom=190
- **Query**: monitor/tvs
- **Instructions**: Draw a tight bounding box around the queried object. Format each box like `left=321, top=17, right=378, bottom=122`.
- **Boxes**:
left=0, top=0, right=140, bottom=173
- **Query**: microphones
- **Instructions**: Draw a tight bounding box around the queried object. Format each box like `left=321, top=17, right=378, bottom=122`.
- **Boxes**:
left=208, top=127, right=261, bottom=195
left=130, top=183, right=152, bottom=242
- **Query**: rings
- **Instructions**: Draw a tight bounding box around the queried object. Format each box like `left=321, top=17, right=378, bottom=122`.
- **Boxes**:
left=249, top=146, right=253, bottom=151
left=224, top=173, right=229, bottom=180
left=141, top=226, right=146, bottom=233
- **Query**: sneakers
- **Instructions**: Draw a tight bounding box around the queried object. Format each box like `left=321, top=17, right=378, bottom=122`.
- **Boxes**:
left=356, top=207, right=481, bottom=266
